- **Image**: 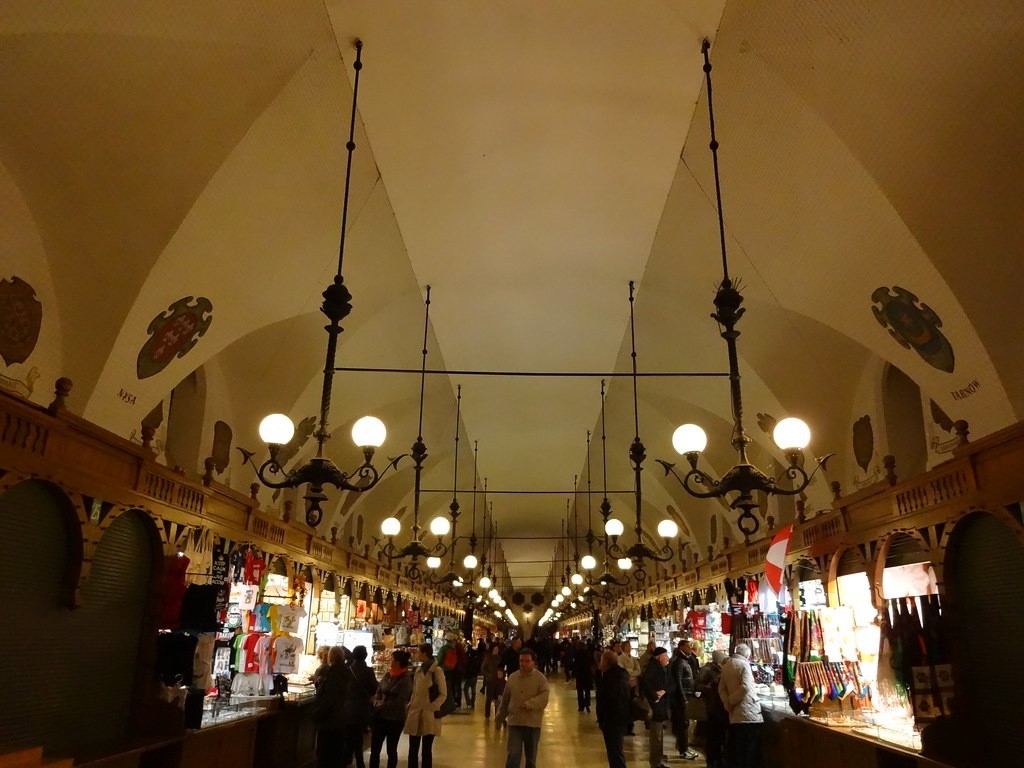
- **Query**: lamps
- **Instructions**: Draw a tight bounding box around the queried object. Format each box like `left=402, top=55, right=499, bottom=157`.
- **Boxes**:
left=652, top=34, right=813, bottom=538
left=426, top=383, right=477, bottom=598
left=538, top=430, right=631, bottom=627
left=255, top=37, right=388, bottom=527
left=380, top=284, right=453, bottom=580
left=603, top=281, right=680, bottom=563
left=460, top=440, right=519, bottom=626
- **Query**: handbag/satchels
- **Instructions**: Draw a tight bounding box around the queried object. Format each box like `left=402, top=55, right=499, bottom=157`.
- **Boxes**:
left=379, top=700, right=409, bottom=720
left=363, top=706, right=380, bottom=722
left=428, top=665, right=457, bottom=718
left=487, top=672, right=507, bottom=701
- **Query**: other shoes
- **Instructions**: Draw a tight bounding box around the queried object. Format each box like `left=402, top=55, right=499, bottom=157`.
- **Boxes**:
left=680, top=752, right=695, bottom=760
left=586, top=704, right=590, bottom=713
left=464, top=705, right=474, bottom=709
left=577, top=708, right=584, bottom=712
left=685, top=749, right=698, bottom=757
left=650, top=763, right=670, bottom=768
left=454, top=706, right=461, bottom=711
left=625, top=730, right=635, bottom=736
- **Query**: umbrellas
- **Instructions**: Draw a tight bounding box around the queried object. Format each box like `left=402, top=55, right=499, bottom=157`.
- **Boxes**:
left=764, top=522, right=820, bottom=597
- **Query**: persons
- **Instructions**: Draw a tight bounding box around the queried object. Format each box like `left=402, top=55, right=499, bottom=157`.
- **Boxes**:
left=403, top=642, right=448, bottom=768
left=308, top=631, right=765, bottom=768
left=596, top=651, right=635, bottom=768
left=495, top=647, right=549, bottom=768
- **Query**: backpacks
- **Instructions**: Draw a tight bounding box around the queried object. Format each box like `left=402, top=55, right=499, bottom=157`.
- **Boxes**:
left=444, top=646, right=458, bottom=671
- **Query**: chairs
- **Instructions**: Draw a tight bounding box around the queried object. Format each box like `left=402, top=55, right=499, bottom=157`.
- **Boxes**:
left=215, top=674, right=234, bottom=697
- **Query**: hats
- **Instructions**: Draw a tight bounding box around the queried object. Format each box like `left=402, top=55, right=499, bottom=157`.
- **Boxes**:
left=653, top=647, right=667, bottom=657
left=442, top=632, right=459, bottom=641
